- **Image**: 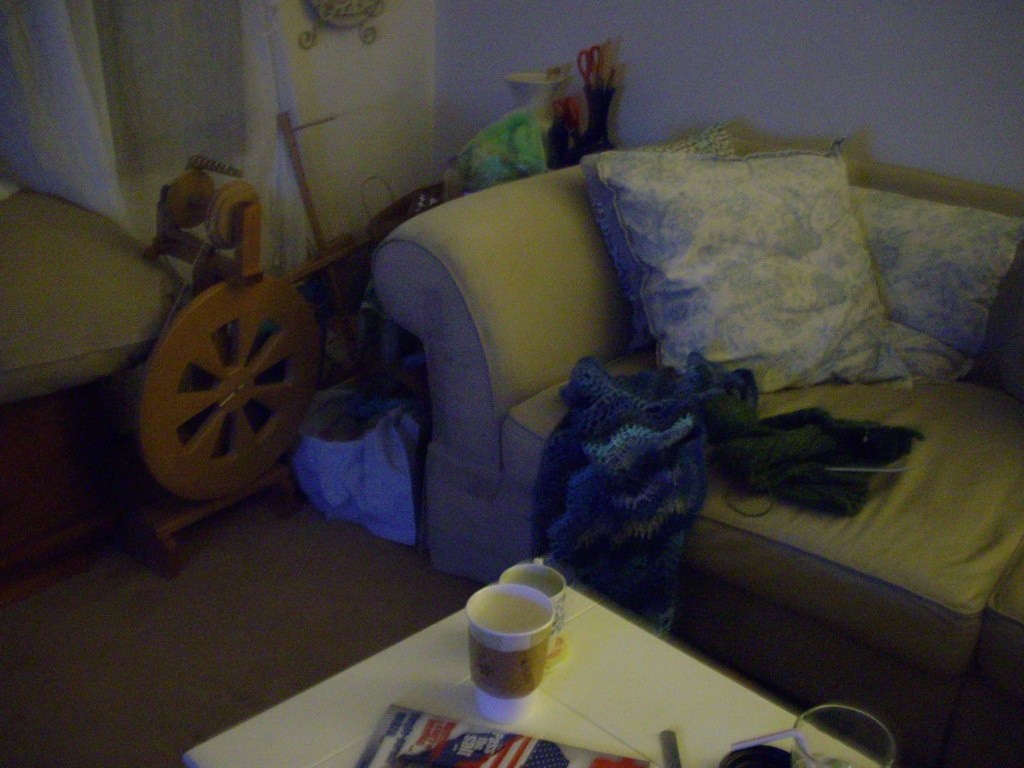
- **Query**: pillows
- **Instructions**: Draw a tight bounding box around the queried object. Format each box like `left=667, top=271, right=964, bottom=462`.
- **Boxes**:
left=0, top=174, right=173, bottom=400
left=580, top=143, right=907, bottom=392
left=856, top=185, right=1024, bottom=383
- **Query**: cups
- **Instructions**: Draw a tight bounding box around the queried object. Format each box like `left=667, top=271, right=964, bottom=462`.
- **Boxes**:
left=465, top=584, right=556, bottom=724
left=497, top=558, right=568, bottom=656
left=792, top=702, right=897, bottom=768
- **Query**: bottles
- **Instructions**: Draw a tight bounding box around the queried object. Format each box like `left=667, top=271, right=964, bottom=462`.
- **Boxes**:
left=548, top=113, right=578, bottom=170
left=504, top=73, right=574, bottom=111
left=579, top=87, right=616, bottom=156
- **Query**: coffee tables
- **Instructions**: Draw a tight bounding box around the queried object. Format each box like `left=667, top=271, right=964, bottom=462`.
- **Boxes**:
left=182, top=588, right=883, bottom=768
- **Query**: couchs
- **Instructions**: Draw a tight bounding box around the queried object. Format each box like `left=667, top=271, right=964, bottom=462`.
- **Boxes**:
left=376, top=131, right=1024, bottom=768
